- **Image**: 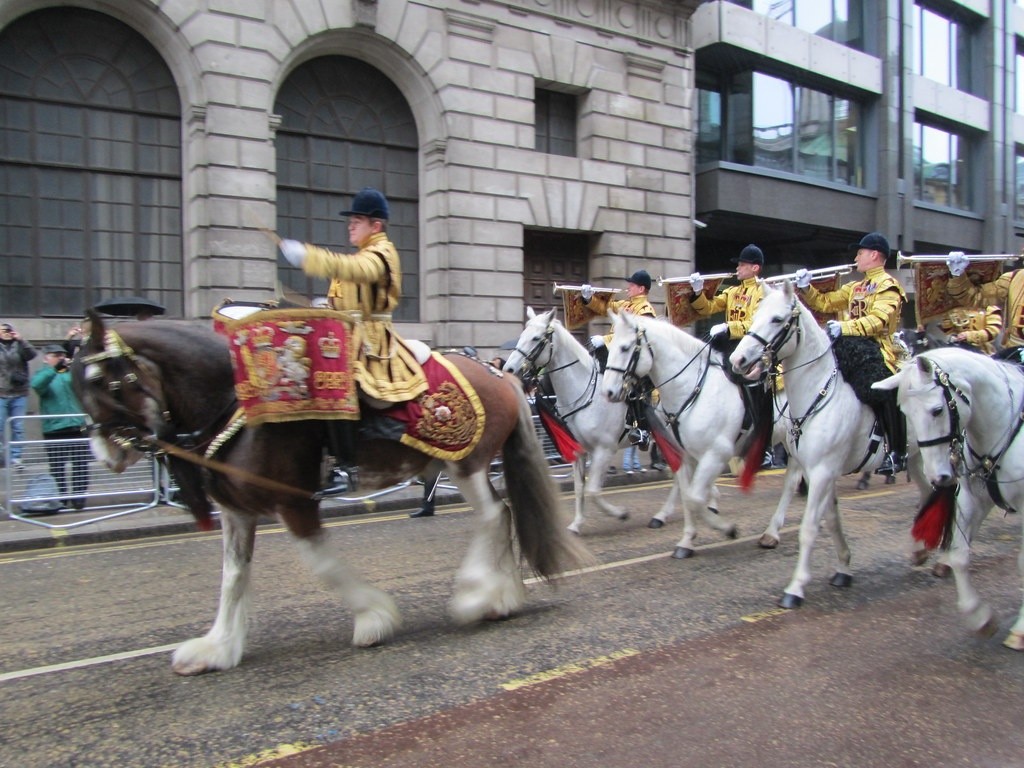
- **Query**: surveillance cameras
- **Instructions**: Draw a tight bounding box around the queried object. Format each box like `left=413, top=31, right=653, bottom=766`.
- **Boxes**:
left=694, top=219, right=707, bottom=229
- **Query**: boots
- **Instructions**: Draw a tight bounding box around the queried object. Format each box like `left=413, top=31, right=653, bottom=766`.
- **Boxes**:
left=875, top=400, right=909, bottom=474
left=314, top=419, right=358, bottom=498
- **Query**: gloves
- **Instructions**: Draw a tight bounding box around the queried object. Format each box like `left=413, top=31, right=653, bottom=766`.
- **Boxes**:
left=689, top=271, right=705, bottom=292
left=312, top=296, right=327, bottom=309
left=796, top=268, right=812, bottom=288
left=946, top=251, right=969, bottom=276
left=827, top=319, right=842, bottom=338
left=581, top=284, right=595, bottom=299
left=709, top=323, right=728, bottom=338
left=279, top=238, right=306, bottom=268
left=590, top=334, right=605, bottom=348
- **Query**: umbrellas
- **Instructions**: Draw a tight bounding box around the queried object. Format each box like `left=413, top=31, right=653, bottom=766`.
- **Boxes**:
left=93, top=296, right=165, bottom=317
left=501, top=339, right=520, bottom=350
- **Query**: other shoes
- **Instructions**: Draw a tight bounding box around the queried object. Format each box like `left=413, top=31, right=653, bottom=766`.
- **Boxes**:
left=624, top=470, right=635, bottom=474
left=650, top=463, right=664, bottom=470
left=633, top=468, right=647, bottom=473
left=9, top=459, right=26, bottom=470
left=72, top=499, right=84, bottom=509
left=608, top=466, right=617, bottom=474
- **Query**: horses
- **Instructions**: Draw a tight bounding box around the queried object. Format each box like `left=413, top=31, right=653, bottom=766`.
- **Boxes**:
left=70, top=275, right=1024, bottom=678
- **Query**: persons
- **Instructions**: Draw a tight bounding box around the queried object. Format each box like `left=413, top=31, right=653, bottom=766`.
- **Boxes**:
left=257, top=186, right=429, bottom=497
left=794, top=231, right=906, bottom=474
left=688, top=243, right=775, bottom=474
left=917, top=306, right=1002, bottom=358
left=945, top=231, right=1024, bottom=348
left=408, top=477, right=436, bottom=518
left=31, top=343, right=89, bottom=508
left=0, top=323, right=38, bottom=468
left=493, top=355, right=667, bottom=475
left=61, top=328, right=89, bottom=359
left=581, top=269, right=656, bottom=451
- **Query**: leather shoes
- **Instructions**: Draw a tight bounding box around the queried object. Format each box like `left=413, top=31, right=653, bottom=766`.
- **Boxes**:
left=629, top=428, right=650, bottom=451
left=410, top=508, right=434, bottom=518
left=760, top=447, right=775, bottom=468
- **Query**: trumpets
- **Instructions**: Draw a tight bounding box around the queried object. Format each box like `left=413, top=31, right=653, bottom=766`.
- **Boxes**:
left=656, top=272, right=740, bottom=287
left=552, top=281, right=629, bottom=296
left=896, top=250, right=1024, bottom=271
left=753, top=263, right=859, bottom=288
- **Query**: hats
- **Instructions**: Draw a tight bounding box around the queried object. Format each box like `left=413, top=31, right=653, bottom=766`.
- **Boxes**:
left=625, top=270, right=651, bottom=288
left=339, top=186, right=390, bottom=219
left=730, top=243, right=764, bottom=264
left=44, top=345, right=67, bottom=353
left=850, top=232, right=890, bottom=257
